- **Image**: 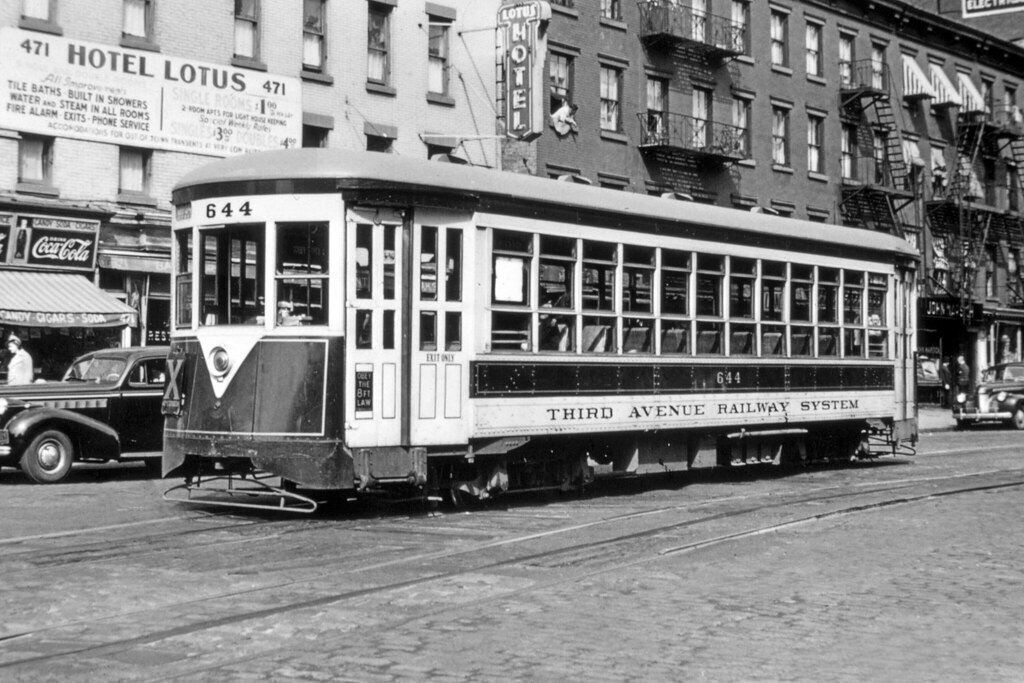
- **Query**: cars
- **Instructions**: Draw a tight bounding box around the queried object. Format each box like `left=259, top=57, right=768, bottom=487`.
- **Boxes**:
left=953, top=362, right=1024, bottom=429
left=0, top=346, right=174, bottom=482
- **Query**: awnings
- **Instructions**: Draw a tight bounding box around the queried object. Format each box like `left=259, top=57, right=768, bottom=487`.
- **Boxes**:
left=0, top=269, right=139, bottom=329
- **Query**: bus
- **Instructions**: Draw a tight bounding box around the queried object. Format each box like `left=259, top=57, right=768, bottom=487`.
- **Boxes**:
left=160, top=153, right=921, bottom=513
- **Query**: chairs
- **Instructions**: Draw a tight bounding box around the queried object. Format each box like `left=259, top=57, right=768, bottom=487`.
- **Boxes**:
left=534, top=324, right=885, bottom=357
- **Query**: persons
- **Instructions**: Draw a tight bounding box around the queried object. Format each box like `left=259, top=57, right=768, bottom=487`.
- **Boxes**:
left=938, top=355, right=970, bottom=408
left=6, top=332, right=34, bottom=385
